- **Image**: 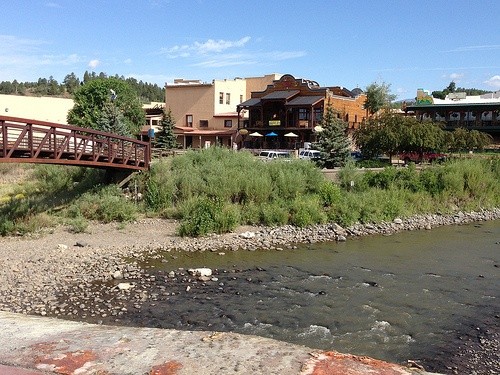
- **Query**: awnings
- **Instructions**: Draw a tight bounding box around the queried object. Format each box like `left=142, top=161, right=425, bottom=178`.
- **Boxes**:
left=265, top=132, right=278, bottom=137
left=284, top=132, right=299, bottom=137
left=249, top=132, right=263, bottom=137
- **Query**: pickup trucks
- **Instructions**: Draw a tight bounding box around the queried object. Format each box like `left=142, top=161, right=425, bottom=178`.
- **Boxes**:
left=400, top=148, right=447, bottom=164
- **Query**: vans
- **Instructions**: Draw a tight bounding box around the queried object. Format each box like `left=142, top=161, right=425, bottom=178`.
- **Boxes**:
left=260, top=149, right=325, bottom=161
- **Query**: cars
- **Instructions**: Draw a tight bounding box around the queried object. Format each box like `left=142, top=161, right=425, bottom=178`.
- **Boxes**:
left=369, top=153, right=390, bottom=164
